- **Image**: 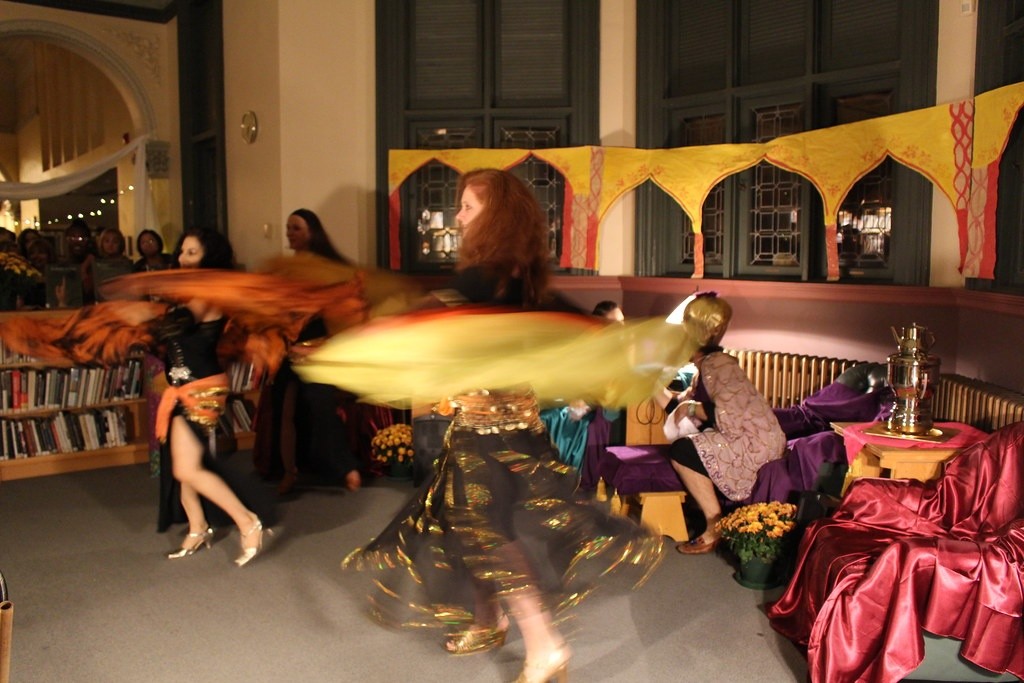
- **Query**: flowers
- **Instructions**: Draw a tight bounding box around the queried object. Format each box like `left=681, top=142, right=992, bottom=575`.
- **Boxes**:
left=714, top=500, right=798, bottom=565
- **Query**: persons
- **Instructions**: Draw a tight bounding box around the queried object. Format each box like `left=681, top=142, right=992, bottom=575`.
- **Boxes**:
left=114, top=226, right=276, bottom=566
left=0, top=214, right=174, bottom=311
left=567, top=300, right=626, bottom=420
left=654, top=294, right=791, bottom=555
left=291, top=166, right=662, bottom=683
left=254, top=208, right=377, bottom=495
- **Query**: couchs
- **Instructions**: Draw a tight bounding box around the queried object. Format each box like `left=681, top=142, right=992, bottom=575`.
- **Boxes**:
left=767, top=421, right=1024, bottom=683
left=595, top=363, right=890, bottom=543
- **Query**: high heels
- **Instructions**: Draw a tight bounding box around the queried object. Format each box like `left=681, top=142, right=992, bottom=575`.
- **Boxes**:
left=165, top=526, right=215, bottom=558
left=234, top=512, right=274, bottom=566
left=514, top=641, right=574, bottom=683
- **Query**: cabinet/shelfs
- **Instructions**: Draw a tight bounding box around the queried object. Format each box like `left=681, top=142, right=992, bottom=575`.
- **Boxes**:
left=0, top=309, right=268, bottom=480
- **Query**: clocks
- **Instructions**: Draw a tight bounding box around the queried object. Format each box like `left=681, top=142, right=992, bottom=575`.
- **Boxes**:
left=240, top=110, right=257, bottom=145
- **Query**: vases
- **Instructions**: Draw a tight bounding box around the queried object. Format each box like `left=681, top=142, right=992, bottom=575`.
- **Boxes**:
left=731, top=551, right=788, bottom=591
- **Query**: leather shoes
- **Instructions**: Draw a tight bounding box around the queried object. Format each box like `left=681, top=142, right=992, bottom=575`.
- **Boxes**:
left=678, top=535, right=725, bottom=554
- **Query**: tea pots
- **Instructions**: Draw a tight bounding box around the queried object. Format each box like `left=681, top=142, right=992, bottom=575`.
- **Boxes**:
left=890, top=323, right=937, bottom=358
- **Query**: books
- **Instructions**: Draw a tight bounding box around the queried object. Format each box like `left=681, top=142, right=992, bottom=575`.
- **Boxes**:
left=0, top=330, right=271, bottom=459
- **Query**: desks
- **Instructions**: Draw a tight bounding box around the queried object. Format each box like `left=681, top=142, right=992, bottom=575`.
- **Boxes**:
left=829, top=417, right=991, bottom=498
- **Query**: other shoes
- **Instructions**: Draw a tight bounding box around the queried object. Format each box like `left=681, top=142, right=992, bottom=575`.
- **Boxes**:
left=443, top=613, right=510, bottom=655
left=276, top=467, right=301, bottom=499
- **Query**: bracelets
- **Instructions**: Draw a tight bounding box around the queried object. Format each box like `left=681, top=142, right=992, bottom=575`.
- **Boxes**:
left=687, top=398, right=696, bottom=417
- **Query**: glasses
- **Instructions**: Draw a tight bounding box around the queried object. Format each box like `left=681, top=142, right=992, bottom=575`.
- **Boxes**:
left=65, top=236, right=88, bottom=244
left=141, top=239, right=156, bottom=245
left=285, top=224, right=301, bottom=231
